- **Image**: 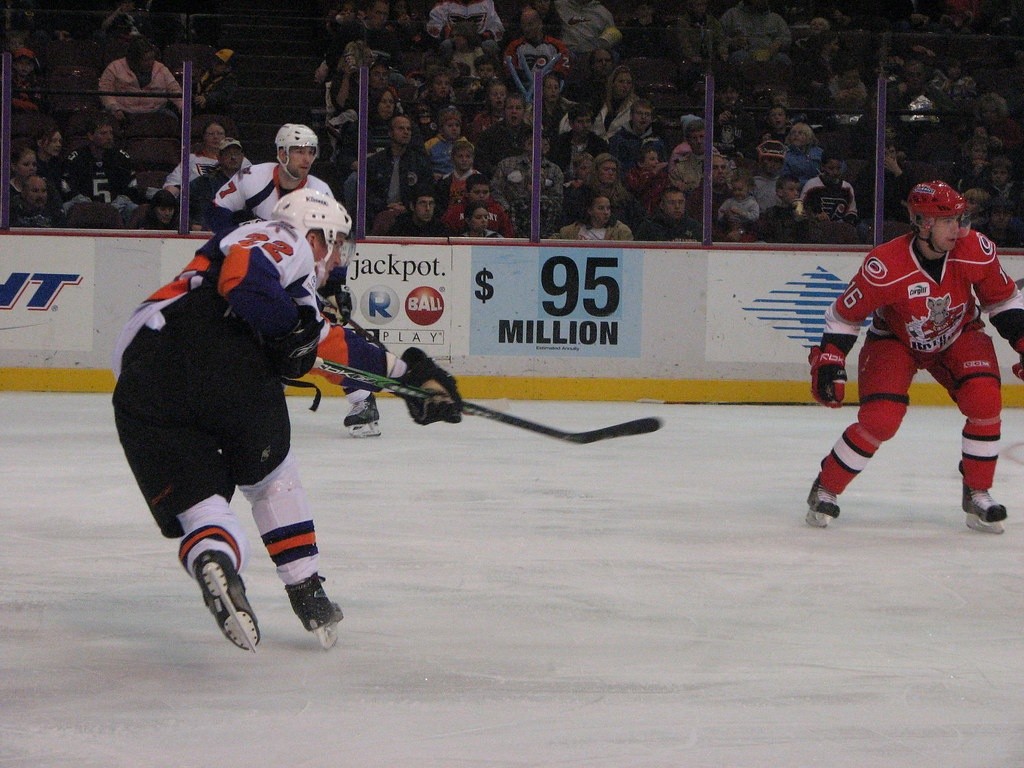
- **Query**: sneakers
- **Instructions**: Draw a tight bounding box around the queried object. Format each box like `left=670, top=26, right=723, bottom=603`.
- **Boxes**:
left=806, top=478, right=841, bottom=529
left=343, top=389, right=382, bottom=438
left=285, top=573, right=344, bottom=650
left=961, top=484, right=1008, bottom=535
left=191, top=550, right=261, bottom=655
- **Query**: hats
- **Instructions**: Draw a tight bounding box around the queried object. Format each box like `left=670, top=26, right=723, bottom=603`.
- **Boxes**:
left=216, top=136, right=243, bottom=152
left=755, top=138, right=787, bottom=162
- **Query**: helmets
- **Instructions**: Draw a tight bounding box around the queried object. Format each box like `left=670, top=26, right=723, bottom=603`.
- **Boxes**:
left=274, top=122, right=318, bottom=167
left=270, top=189, right=352, bottom=262
left=906, top=180, right=969, bottom=236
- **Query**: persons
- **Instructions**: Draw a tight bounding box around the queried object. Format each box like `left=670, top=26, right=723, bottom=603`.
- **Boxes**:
left=112, top=188, right=463, bottom=651
left=213, top=121, right=380, bottom=426
left=0, top=0, right=1024, bottom=243
left=807, top=182, right=1024, bottom=523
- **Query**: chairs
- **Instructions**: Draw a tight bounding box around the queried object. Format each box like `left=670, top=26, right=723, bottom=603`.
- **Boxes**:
left=15, top=0, right=1024, bottom=241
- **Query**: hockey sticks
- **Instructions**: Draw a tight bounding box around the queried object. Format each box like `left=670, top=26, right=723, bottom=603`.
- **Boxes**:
left=318, top=358, right=663, bottom=445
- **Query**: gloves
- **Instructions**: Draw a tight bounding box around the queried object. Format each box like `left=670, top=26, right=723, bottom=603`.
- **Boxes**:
left=808, top=344, right=848, bottom=409
left=400, top=348, right=463, bottom=426
left=283, top=305, right=321, bottom=379
left=1011, top=334, right=1024, bottom=379
